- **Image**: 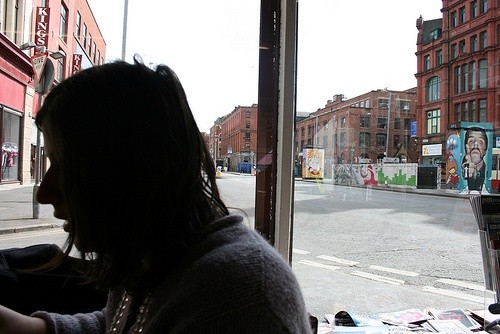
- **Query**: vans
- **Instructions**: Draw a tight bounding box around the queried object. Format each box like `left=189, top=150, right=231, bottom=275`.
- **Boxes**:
left=237, top=162, right=255, bottom=174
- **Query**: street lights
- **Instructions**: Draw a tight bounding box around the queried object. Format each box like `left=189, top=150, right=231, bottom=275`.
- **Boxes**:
left=19, top=41, right=66, bottom=219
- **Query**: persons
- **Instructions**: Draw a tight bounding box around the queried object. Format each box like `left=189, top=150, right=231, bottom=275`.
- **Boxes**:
left=0, top=52, right=313, bottom=334
left=461, top=127, right=488, bottom=191
